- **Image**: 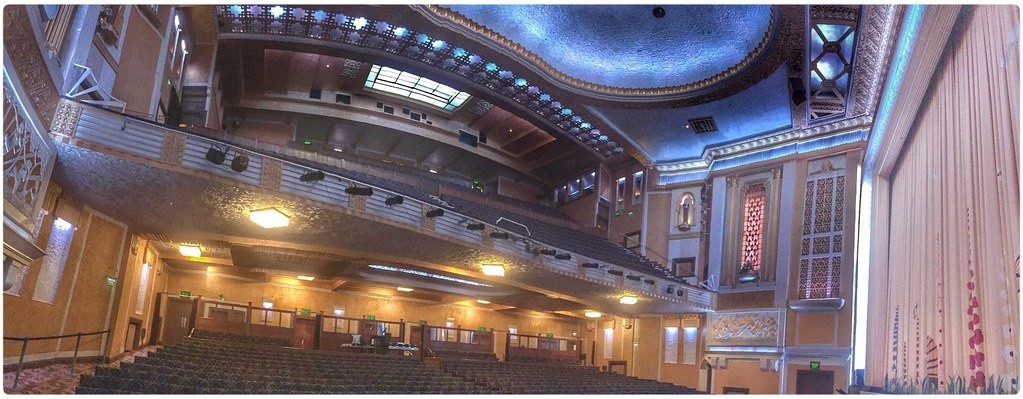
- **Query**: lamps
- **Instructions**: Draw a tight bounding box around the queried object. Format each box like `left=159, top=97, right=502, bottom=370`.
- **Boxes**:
left=582, top=260, right=598, bottom=268
left=426, top=206, right=444, bottom=217
left=626, top=272, right=640, bottom=281
left=490, top=228, right=509, bottom=239
left=600, top=265, right=623, bottom=276
left=738, top=263, right=760, bottom=282
left=300, top=168, right=325, bottom=184
left=385, top=193, right=404, bottom=209
left=231, top=148, right=249, bottom=173
left=667, top=285, right=676, bottom=294
left=540, top=246, right=572, bottom=260
left=206, top=143, right=231, bottom=165
left=344, top=182, right=374, bottom=197
left=644, top=280, right=655, bottom=285
left=459, top=219, right=485, bottom=230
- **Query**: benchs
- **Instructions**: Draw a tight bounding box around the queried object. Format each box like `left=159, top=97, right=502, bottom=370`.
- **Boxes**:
left=75, top=329, right=712, bottom=395
